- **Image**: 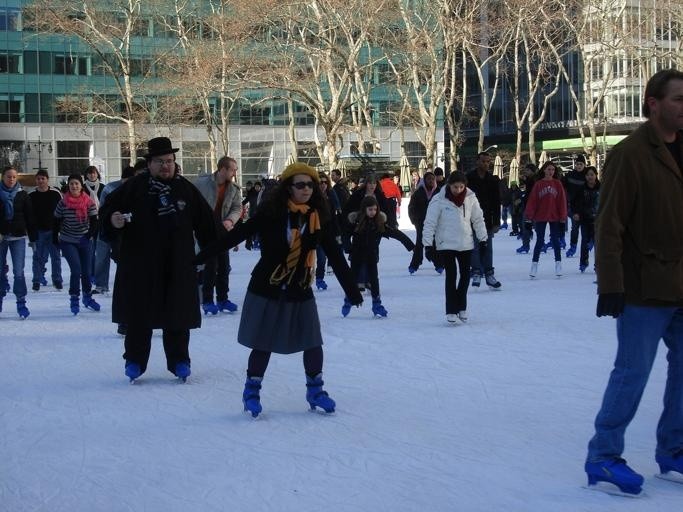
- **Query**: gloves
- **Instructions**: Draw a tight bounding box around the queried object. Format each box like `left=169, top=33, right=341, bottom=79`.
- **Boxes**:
left=597, top=292, right=624, bottom=318
left=425, top=246, right=434, bottom=262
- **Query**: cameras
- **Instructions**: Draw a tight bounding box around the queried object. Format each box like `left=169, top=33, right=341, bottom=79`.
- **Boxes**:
left=122, top=212, right=133, bottom=222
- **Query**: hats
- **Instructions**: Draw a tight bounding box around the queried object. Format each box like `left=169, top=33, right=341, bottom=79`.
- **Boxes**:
left=576, top=154, right=585, bottom=162
left=142, top=137, right=179, bottom=158
left=281, top=162, right=321, bottom=184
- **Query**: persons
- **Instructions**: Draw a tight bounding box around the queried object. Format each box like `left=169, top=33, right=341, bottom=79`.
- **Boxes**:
left=192, top=156, right=279, bottom=316
left=507, top=156, right=603, bottom=278
left=406, top=152, right=508, bottom=323
left=0, top=160, right=149, bottom=321
left=194, top=160, right=364, bottom=417
left=313, top=167, right=418, bottom=320
left=97, top=135, right=222, bottom=385
left=584, top=69, right=682, bottom=494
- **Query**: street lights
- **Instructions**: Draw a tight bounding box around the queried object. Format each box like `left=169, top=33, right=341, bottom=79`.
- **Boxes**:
left=196, top=164, right=201, bottom=176
left=26, top=136, right=53, bottom=175
left=481, top=144, right=498, bottom=154
left=0, top=143, right=14, bottom=168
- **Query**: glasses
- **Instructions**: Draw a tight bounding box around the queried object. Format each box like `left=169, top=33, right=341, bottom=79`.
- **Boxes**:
left=292, top=182, right=314, bottom=190
left=153, top=159, right=174, bottom=165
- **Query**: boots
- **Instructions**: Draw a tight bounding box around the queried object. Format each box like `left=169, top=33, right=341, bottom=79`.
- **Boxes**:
left=306, top=371, right=336, bottom=413
left=243, top=376, right=262, bottom=413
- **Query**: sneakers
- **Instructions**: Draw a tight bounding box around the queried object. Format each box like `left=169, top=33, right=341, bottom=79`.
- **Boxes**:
left=217, top=300, right=237, bottom=311
left=459, top=311, right=468, bottom=320
left=17, top=296, right=29, bottom=317
left=472, top=274, right=481, bottom=287
left=585, top=458, right=644, bottom=489
left=316, top=266, right=333, bottom=289
left=203, top=304, right=218, bottom=314
left=655, top=450, right=683, bottom=473
left=342, top=282, right=387, bottom=317
left=409, top=265, right=416, bottom=273
left=33, top=277, right=108, bottom=313
left=486, top=276, right=501, bottom=288
left=436, top=267, right=444, bottom=273
left=447, top=314, right=457, bottom=322
left=175, top=363, right=190, bottom=378
left=501, top=223, right=586, bottom=277
left=125, top=363, right=140, bottom=378
